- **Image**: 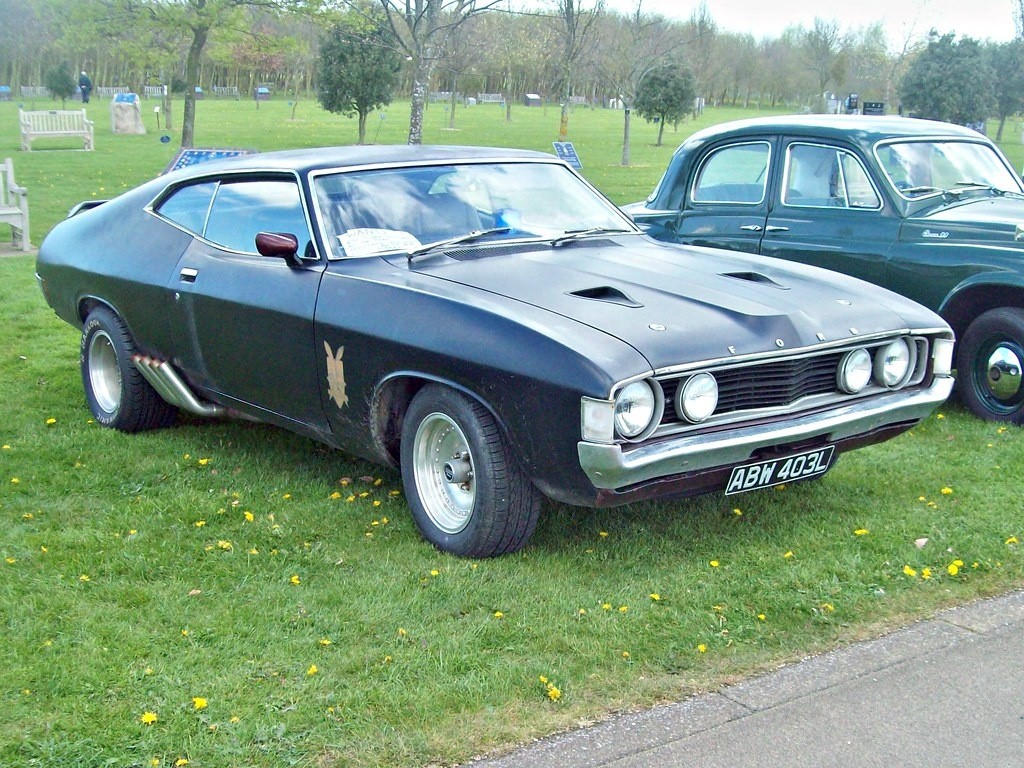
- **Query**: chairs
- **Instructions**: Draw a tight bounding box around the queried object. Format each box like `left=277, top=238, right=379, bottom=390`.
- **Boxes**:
left=414, top=192, right=484, bottom=244
left=0, top=158, right=30, bottom=252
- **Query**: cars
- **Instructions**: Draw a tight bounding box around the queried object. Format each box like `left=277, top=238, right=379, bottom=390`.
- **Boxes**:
left=617, top=111, right=1024, bottom=424
left=33, top=144, right=959, bottom=560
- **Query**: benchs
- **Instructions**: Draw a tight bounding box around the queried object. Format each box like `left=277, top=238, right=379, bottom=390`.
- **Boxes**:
left=21, top=107, right=94, bottom=151
left=569, top=96, right=589, bottom=108
left=97, top=86, right=130, bottom=98
left=477, top=92, right=505, bottom=105
left=693, top=183, right=802, bottom=205
left=430, top=92, right=452, bottom=103
left=143, top=86, right=162, bottom=101
left=215, top=86, right=240, bottom=101
left=21, top=85, right=52, bottom=102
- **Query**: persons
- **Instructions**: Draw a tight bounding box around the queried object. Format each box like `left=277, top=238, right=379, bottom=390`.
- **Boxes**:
left=80, top=71, right=91, bottom=104
